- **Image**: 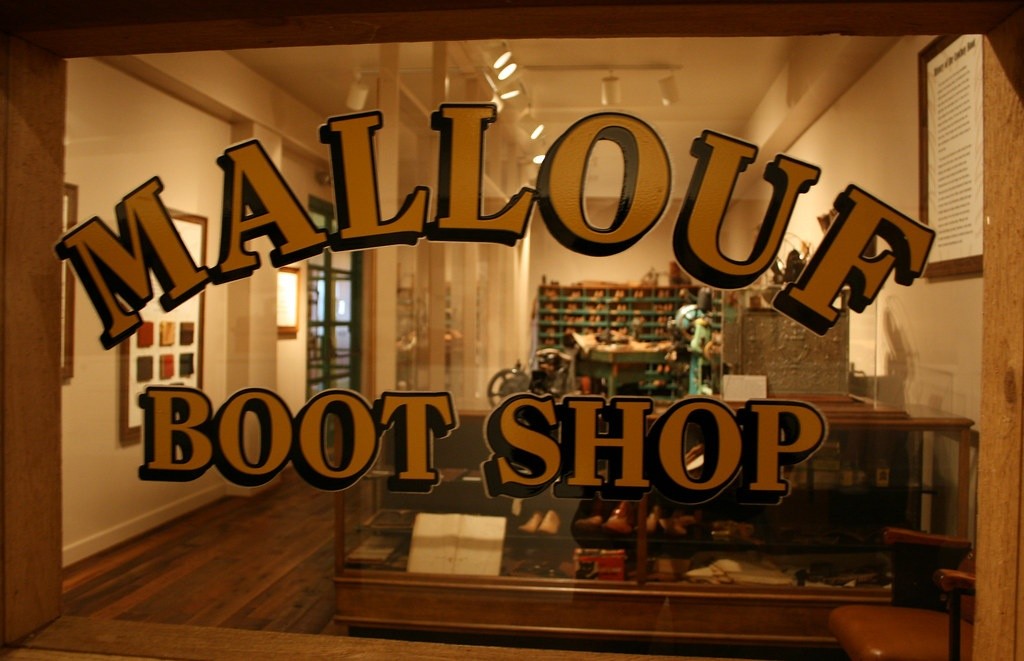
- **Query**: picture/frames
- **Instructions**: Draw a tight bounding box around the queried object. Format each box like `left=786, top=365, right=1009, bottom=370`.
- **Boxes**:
left=114, top=200, right=212, bottom=444
left=271, top=260, right=301, bottom=344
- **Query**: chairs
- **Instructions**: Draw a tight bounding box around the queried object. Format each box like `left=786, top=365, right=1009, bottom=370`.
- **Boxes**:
left=828, top=523, right=976, bottom=661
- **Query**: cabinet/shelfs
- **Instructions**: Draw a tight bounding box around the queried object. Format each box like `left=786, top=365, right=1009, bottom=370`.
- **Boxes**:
left=533, top=277, right=700, bottom=399
left=328, top=390, right=974, bottom=648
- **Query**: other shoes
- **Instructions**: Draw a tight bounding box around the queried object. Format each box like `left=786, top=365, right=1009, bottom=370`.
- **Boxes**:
left=518, top=508, right=561, bottom=535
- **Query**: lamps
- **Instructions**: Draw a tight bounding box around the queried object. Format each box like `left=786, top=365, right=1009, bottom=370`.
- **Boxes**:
left=528, top=143, right=547, bottom=165
left=519, top=112, right=544, bottom=140
left=481, top=45, right=523, bottom=100
